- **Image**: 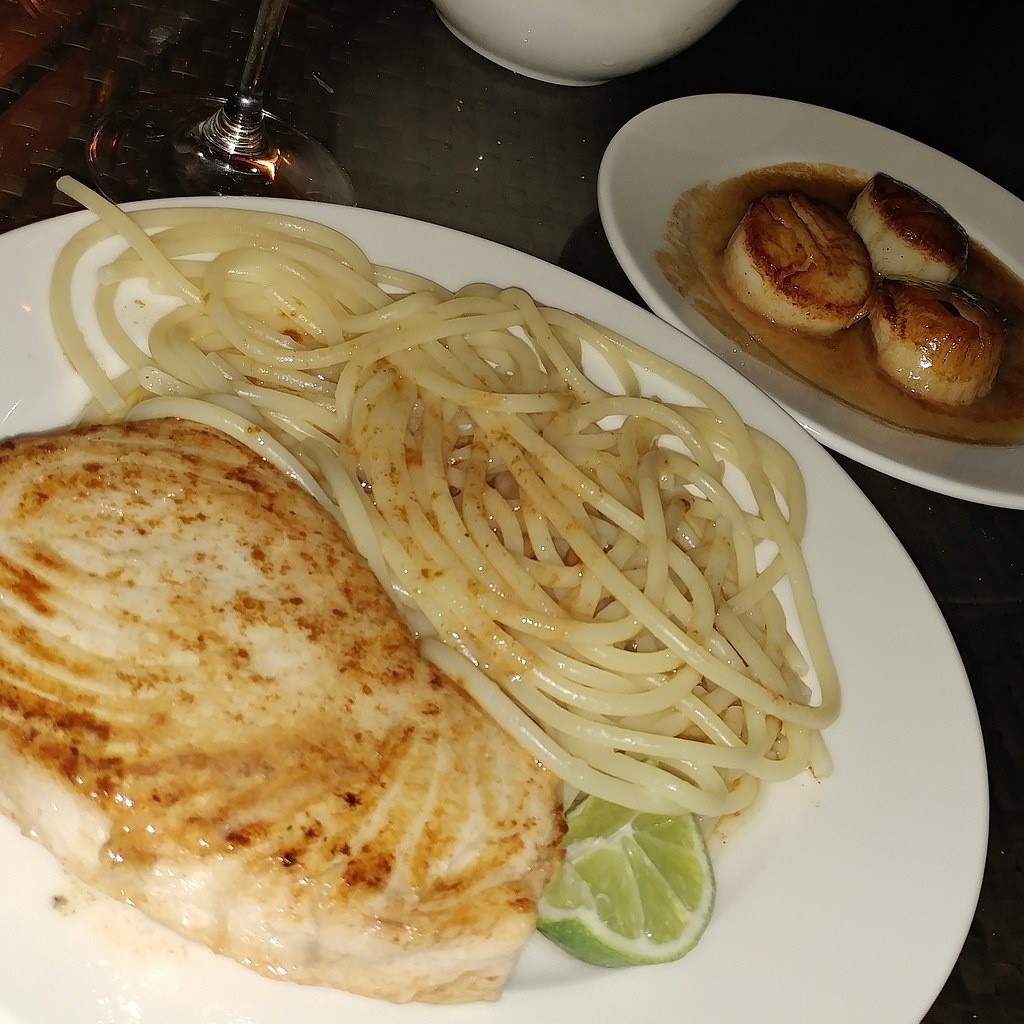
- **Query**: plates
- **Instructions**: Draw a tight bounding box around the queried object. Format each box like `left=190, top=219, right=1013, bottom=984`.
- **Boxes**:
left=597, top=92, right=1023, bottom=517
left=1, top=192, right=988, bottom=1024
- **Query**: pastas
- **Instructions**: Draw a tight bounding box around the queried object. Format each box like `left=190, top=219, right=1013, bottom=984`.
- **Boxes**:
left=52, top=176, right=840, bottom=833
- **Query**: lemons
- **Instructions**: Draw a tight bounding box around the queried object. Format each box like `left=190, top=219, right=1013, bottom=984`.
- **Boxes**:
left=529, top=762, right=716, bottom=967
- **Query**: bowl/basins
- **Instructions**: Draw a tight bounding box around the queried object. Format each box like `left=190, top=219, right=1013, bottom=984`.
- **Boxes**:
left=434, top=0, right=741, bottom=86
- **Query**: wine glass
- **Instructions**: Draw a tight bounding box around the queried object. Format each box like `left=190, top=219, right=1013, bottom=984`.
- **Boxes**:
left=86, top=0, right=358, bottom=216
